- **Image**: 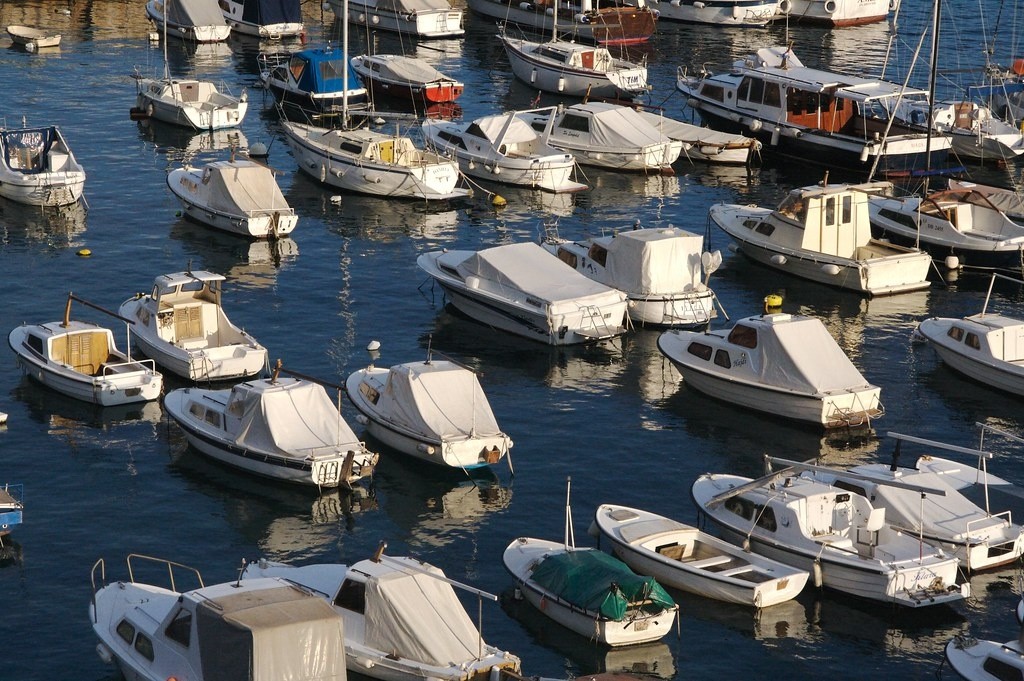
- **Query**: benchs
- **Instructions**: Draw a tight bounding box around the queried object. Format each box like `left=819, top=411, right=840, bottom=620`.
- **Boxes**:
left=686, top=554, right=734, bottom=568
left=716, top=564, right=757, bottom=578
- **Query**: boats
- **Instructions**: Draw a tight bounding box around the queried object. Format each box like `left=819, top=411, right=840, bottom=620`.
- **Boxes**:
left=345, top=335, right=510, bottom=471
left=913, top=273, right=1024, bottom=395
left=588, top=503, right=811, bottom=608
left=7, top=292, right=163, bottom=406
left=176, top=439, right=381, bottom=567
left=503, top=476, right=682, bottom=646
left=498, top=585, right=677, bottom=680
left=916, top=421, right=1024, bottom=525
left=353, top=427, right=513, bottom=565
left=798, top=432, right=1024, bottom=575
left=656, top=295, right=885, bottom=429
left=931, top=591, right=1024, bottom=681
left=1, top=1, right=1023, bottom=347
left=690, top=453, right=973, bottom=610
left=162, top=359, right=379, bottom=488
left=237, top=537, right=522, bottom=680
left=87, top=553, right=349, bottom=681
left=666, top=585, right=815, bottom=641
left=117, top=260, right=269, bottom=382
